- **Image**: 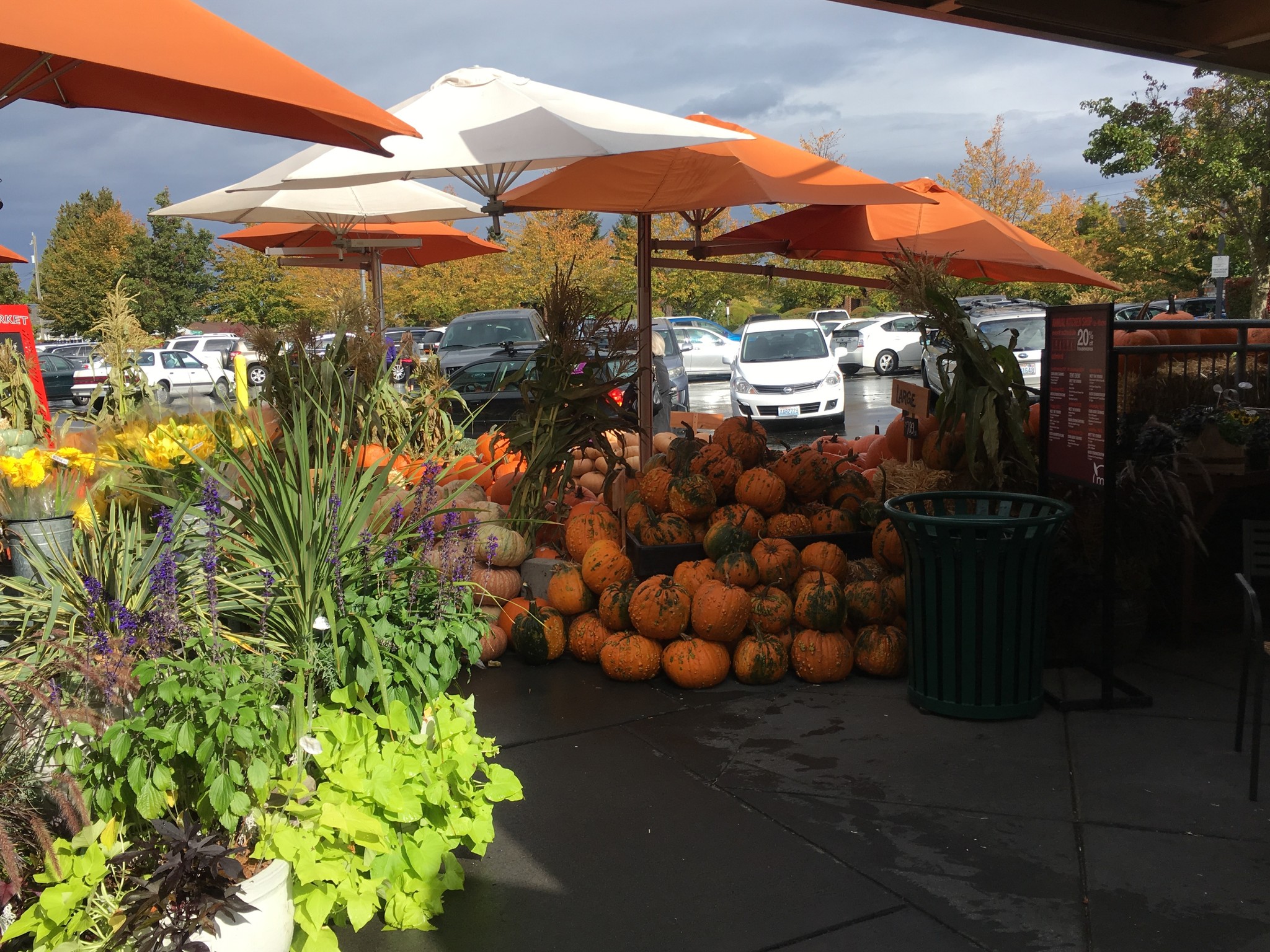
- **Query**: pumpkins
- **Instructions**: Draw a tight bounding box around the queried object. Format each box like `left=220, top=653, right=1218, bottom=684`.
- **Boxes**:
left=298, top=405, right=1039, bottom=690
left=1111, top=292, right=1270, bottom=372
left=0, top=404, right=283, bottom=501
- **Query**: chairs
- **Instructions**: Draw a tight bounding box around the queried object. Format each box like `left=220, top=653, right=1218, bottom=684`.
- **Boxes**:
left=1233, top=515, right=1270, bottom=803
left=749, top=333, right=817, bottom=357
left=461, top=320, right=527, bottom=345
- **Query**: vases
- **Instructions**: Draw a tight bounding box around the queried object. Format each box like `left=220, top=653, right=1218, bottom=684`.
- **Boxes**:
left=1244, top=448, right=1270, bottom=471
left=161, top=501, right=216, bottom=564
left=160, top=852, right=297, bottom=952
left=0, top=510, right=75, bottom=643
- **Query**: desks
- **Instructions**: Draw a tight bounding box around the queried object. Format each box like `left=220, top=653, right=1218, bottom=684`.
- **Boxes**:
left=1121, top=471, right=1270, bottom=650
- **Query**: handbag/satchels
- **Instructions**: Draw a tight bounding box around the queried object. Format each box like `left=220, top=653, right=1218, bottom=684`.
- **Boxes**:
left=411, top=359, right=417, bottom=368
left=633, top=363, right=663, bottom=413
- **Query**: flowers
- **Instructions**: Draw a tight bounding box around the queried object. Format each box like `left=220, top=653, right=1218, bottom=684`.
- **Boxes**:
left=1213, top=404, right=1270, bottom=453
left=0, top=327, right=526, bottom=952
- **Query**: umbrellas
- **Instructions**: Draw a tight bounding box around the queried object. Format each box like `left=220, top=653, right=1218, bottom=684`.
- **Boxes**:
left=147, top=179, right=506, bottom=261
left=227, top=64, right=756, bottom=235
left=0, top=245, right=29, bottom=263
left=496, top=112, right=940, bottom=466
left=217, top=221, right=508, bottom=374
left=0, top=0, right=423, bottom=158
left=693, top=175, right=1123, bottom=291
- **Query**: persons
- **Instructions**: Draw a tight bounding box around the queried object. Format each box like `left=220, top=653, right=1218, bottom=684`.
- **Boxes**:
left=399, top=331, right=420, bottom=392
left=385, top=335, right=396, bottom=375
left=622, top=331, right=671, bottom=436
